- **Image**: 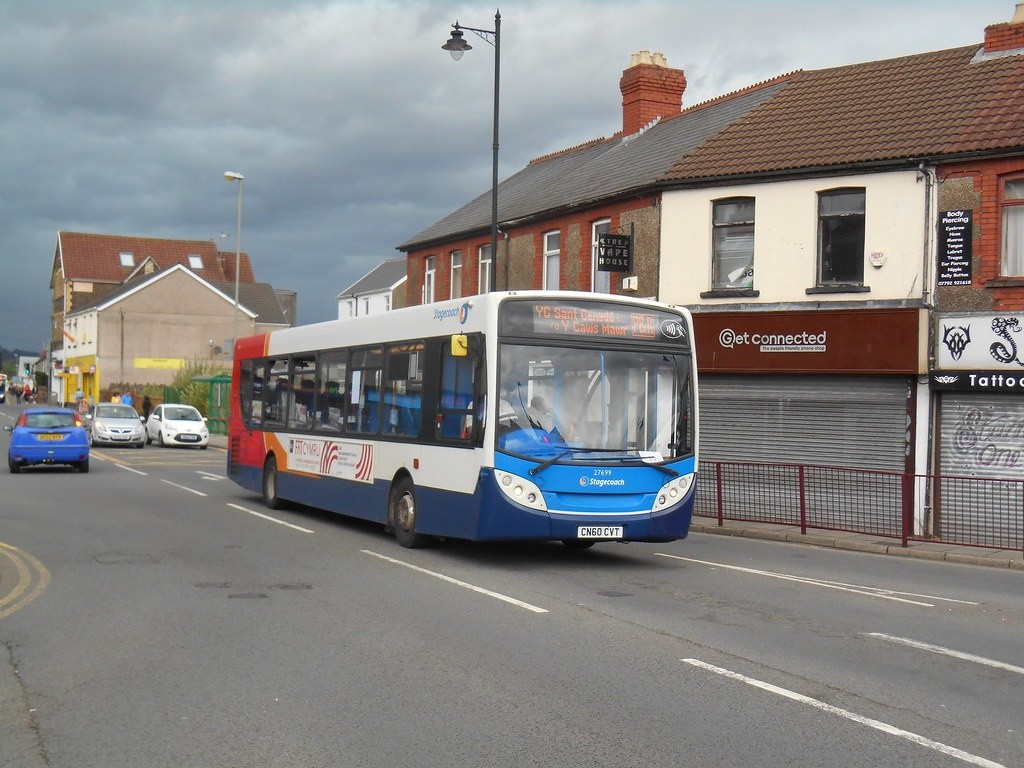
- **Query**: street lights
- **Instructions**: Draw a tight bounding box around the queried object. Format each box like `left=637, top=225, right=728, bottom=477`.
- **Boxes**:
left=223, top=170, right=247, bottom=353
left=440, top=5, right=502, bottom=292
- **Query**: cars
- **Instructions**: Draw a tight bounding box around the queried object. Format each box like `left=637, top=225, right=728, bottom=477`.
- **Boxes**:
left=3, top=406, right=91, bottom=474
left=0, top=387, right=7, bottom=403
left=146, top=403, right=211, bottom=449
left=85, top=403, right=149, bottom=449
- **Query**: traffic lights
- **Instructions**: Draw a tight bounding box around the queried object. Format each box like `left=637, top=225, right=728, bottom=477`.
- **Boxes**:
left=25, top=367, right=30, bottom=374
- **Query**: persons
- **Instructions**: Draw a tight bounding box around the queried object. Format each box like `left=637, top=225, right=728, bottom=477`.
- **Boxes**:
left=142, top=395, right=151, bottom=423
left=8, top=382, right=89, bottom=415
left=111, top=390, right=135, bottom=406
left=465, top=396, right=518, bottom=436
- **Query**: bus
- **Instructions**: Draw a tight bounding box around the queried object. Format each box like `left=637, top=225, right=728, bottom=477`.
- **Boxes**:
left=226, top=288, right=702, bottom=549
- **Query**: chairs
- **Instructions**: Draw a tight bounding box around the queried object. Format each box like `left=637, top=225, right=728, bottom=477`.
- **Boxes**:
left=251, top=377, right=472, bottom=439
